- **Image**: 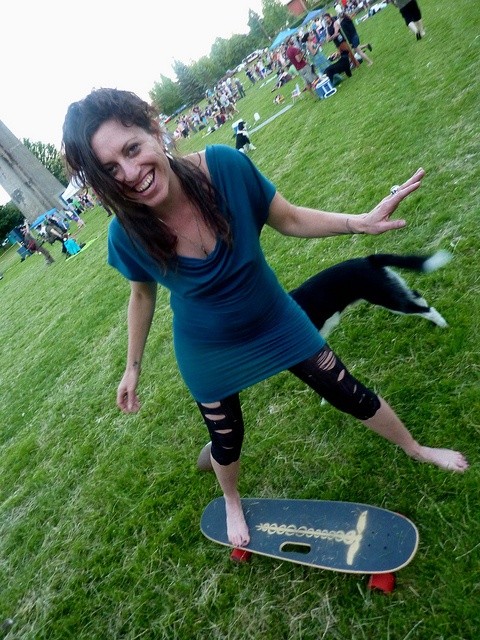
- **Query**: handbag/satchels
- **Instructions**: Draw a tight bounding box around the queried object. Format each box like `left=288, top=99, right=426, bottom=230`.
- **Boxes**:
left=23, top=237, right=35, bottom=251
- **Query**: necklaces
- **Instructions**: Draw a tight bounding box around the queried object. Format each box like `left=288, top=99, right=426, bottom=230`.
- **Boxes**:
left=156, top=198, right=210, bottom=257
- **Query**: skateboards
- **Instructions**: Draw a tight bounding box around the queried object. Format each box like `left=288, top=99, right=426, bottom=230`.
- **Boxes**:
left=200, top=497, right=419, bottom=592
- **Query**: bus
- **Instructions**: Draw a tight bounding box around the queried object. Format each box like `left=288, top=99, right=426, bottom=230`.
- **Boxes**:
left=241, top=52, right=259, bottom=65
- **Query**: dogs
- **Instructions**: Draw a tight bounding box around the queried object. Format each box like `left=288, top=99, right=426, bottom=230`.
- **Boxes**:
left=235, top=121, right=256, bottom=153
left=221, top=102, right=240, bottom=121
left=287, top=248, right=457, bottom=408
left=323, top=50, right=352, bottom=85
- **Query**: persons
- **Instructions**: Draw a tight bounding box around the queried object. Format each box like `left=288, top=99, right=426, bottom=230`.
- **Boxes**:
left=173, top=76, right=248, bottom=141
left=20, top=221, right=55, bottom=264
left=61, top=87, right=471, bottom=546
left=389, top=0, right=426, bottom=39
left=245, top=0, right=388, bottom=86
left=43, top=207, right=84, bottom=256
left=76, top=181, right=113, bottom=217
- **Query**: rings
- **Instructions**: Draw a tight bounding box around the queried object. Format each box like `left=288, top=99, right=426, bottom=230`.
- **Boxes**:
left=391, top=185, right=400, bottom=194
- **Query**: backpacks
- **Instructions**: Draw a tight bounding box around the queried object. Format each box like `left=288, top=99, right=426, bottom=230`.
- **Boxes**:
left=64, top=240, right=81, bottom=254
